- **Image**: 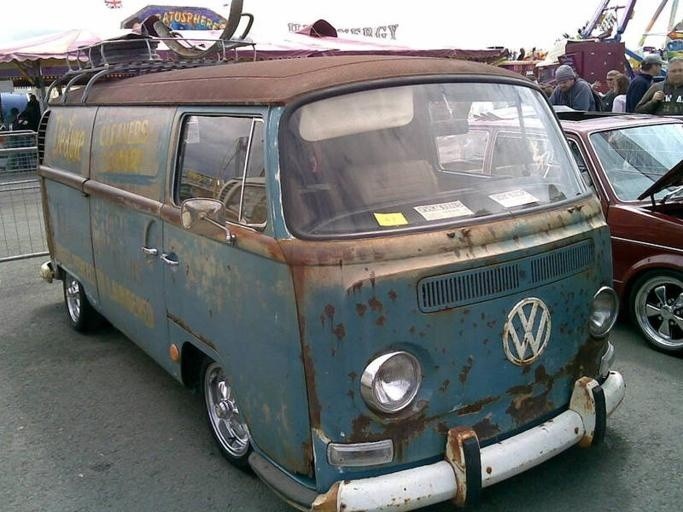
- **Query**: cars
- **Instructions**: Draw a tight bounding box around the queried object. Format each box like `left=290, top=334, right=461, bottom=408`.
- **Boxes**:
left=434, top=109, right=683, bottom=356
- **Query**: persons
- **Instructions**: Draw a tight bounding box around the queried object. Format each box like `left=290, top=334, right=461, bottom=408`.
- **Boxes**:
left=548, top=54, right=683, bottom=115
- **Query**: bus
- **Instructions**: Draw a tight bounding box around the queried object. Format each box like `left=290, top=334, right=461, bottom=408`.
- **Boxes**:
left=31, top=35, right=628, bottom=512
